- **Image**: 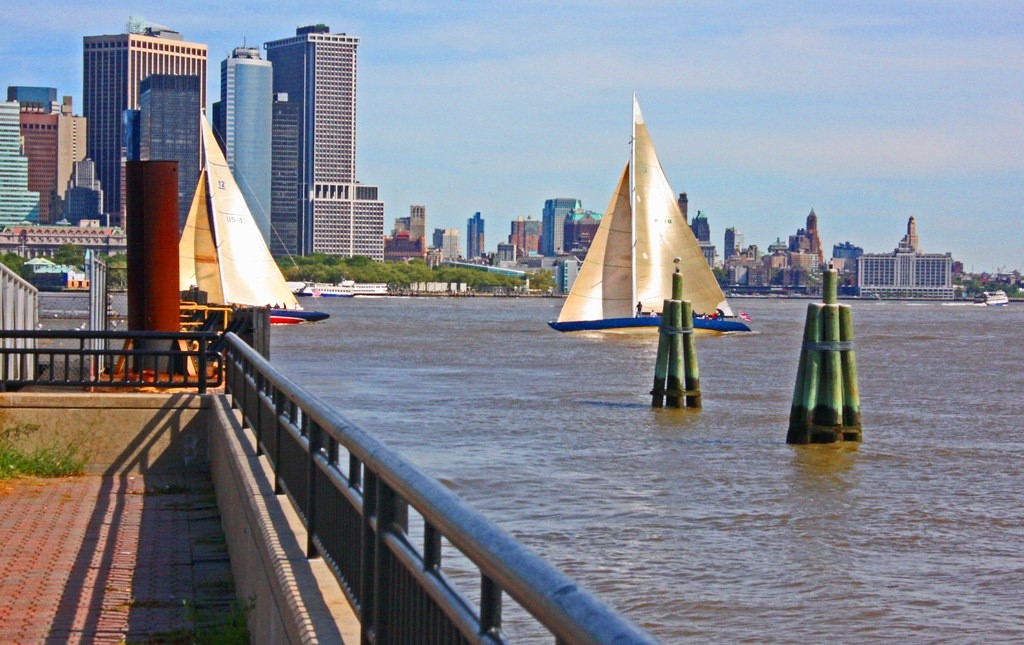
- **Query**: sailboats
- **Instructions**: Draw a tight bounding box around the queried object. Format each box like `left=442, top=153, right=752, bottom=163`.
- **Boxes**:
left=546, top=91, right=754, bottom=339
left=179, top=107, right=332, bottom=324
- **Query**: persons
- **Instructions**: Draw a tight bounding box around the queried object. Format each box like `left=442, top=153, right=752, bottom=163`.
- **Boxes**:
left=636, top=302, right=642, bottom=318
left=703, top=313, right=710, bottom=320
left=692, top=310, right=697, bottom=318
left=650, top=309, right=657, bottom=317
left=716, top=308, right=724, bottom=321
left=712, top=312, right=717, bottom=320
left=265, top=303, right=272, bottom=310
left=273, top=303, right=280, bottom=309
left=282, top=303, right=287, bottom=309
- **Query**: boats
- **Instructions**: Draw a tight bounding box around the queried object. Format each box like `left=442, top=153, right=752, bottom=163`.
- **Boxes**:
left=286, top=279, right=388, bottom=298
left=974, top=290, right=1010, bottom=307
